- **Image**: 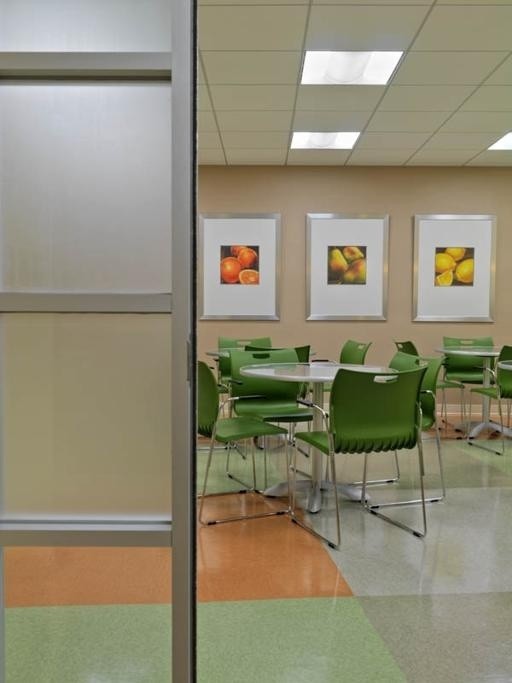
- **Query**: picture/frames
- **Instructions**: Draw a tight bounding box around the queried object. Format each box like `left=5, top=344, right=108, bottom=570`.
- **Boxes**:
left=197, top=211, right=279, bottom=321
left=412, top=214, right=496, bottom=323
left=304, top=212, right=386, bottom=322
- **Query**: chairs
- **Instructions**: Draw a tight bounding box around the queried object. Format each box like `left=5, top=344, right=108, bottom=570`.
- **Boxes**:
left=196, top=361, right=291, bottom=527
left=289, top=366, right=428, bottom=550
left=339, top=340, right=373, bottom=364
left=217, top=337, right=272, bottom=418
left=468, top=344, right=511, bottom=455
left=352, top=352, right=445, bottom=509
left=244, top=343, right=312, bottom=445
left=439, top=336, right=496, bottom=431
left=227, top=347, right=313, bottom=491
left=395, top=339, right=468, bottom=441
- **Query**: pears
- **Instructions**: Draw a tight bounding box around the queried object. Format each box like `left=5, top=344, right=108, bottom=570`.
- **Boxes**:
left=328, top=246, right=367, bottom=284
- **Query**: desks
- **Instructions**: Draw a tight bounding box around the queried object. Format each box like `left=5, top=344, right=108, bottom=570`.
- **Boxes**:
left=206, top=351, right=317, bottom=450
left=435, top=346, right=503, bottom=438
left=240, top=362, right=400, bottom=514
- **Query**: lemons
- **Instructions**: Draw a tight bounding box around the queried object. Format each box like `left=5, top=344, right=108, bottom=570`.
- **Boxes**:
left=434, top=247, right=474, bottom=287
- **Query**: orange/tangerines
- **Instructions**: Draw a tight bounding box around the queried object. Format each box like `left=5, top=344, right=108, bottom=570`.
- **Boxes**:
left=221, top=246, right=259, bottom=284
